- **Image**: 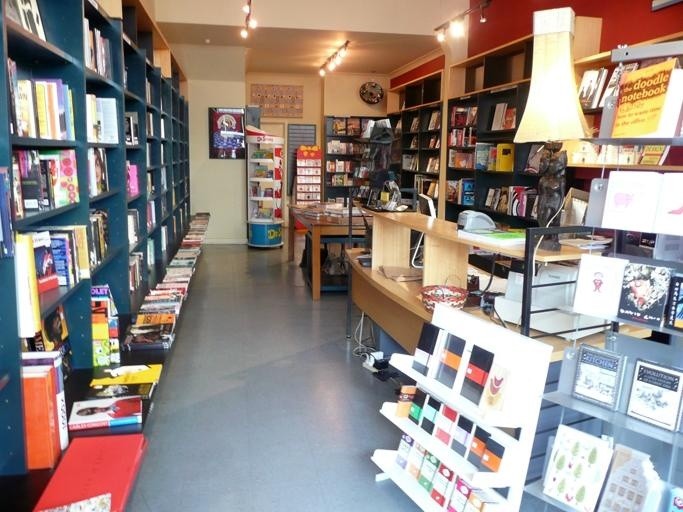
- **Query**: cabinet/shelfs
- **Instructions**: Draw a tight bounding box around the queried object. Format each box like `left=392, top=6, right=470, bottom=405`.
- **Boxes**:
left=345, top=207, right=654, bottom=371
left=0, top=0, right=189, bottom=512
left=366, top=303, right=554, bottom=512
left=319, top=115, right=377, bottom=205
left=384, top=99, right=446, bottom=218
left=516, top=39, right=683, bottom=511
left=445, top=77, right=544, bottom=279
left=451, top=16, right=604, bottom=100
left=245, top=133, right=285, bottom=248
left=294, top=142, right=324, bottom=235
left=385, top=69, right=445, bottom=114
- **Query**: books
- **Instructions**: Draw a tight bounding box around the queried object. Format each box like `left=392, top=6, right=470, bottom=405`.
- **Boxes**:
left=394, top=321, right=513, bottom=512
left=4, top=0, right=164, bottom=146
left=287, top=102, right=543, bottom=248
left=541, top=55, right=682, bottom=512
left=9, top=142, right=210, bottom=512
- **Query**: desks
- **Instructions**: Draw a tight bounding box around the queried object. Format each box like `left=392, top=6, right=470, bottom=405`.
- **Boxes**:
left=28, top=211, right=212, bottom=512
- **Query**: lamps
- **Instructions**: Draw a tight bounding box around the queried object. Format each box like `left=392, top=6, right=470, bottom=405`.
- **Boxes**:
left=477, top=4, right=489, bottom=23
left=318, top=43, right=353, bottom=78
left=514, top=5, right=595, bottom=250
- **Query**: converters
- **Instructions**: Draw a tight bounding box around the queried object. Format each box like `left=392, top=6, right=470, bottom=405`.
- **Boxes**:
left=374, top=359, right=388, bottom=369
left=369, top=351, right=384, bottom=366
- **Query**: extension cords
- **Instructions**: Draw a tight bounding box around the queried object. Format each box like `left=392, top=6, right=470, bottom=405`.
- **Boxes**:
left=361, top=362, right=382, bottom=372
